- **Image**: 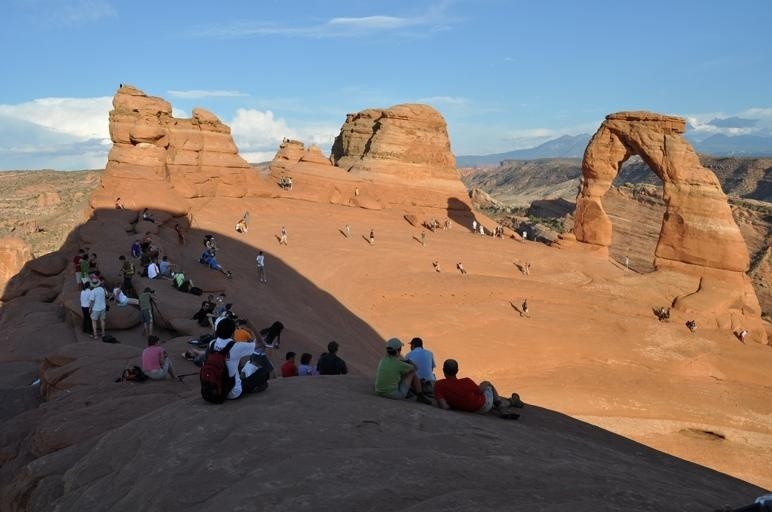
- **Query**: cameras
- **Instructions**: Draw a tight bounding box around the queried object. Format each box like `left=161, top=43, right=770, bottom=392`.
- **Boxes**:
left=239, top=320, right=247, bottom=325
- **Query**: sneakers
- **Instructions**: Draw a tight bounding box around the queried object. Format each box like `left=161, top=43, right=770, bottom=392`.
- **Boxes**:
left=415, top=392, right=433, bottom=406
left=498, top=406, right=522, bottom=420
left=512, top=392, right=525, bottom=409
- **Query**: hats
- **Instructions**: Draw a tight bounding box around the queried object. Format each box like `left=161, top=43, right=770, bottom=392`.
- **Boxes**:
left=215, top=318, right=235, bottom=339
left=385, top=337, right=405, bottom=352
left=90, top=279, right=101, bottom=288
left=408, top=337, right=423, bottom=348
left=233, top=328, right=251, bottom=342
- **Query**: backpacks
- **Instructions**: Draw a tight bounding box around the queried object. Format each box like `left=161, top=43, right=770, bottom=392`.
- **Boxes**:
left=121, top=365, right=150, bottom=383
left=199, top=340, right=237, bottom=406
left=124, top=263, right=136, bottom=276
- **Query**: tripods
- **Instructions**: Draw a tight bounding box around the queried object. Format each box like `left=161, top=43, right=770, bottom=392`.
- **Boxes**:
left=144, top=298, right=173, bottom=336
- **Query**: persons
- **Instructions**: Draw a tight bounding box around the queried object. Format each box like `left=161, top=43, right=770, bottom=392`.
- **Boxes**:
left=141, top=336, right=185, bottom=382
left=344, top=224, right=352, bottom=236
left=624, top=255, right=630, bottom=272
left=370, top=228, right=375, bottom=243
left=424, top=216, right=452, bottom=232
left=297, top=353, right=313, bottom=376
left=176, top=223, right=186, bottom=246
left=281, top=352, right=299, bottom=377
left=116, top=198, right=128, bottom=214
left=235, top=212, right=248, bottom=234
left=312, top=352, right=328, bottom=376
left=404, top=338, right=436, bottom=398
left=520, top=299, right=528, bottom=318
left=653, top=306, right=697, bottom=335
left=142, top=208, right=155, bottom=224
left=421, top=231, right=425, bottom=247
left=739, top=330, right=748, bottom=343
left=198, top=234, right=232, bottom=280
left=523, top=262, right=528, bottom=275
left=181, top=293, right=284, bottom=401
left=131, top=231, right=204, bottom=296
left=278, top=177, right=293, bottom=191
left=432, top=359, right=524, bottom=418
left=280, top=226, right=288, bottom=246
left=458, top=262, right=466, bottom=274
left=256, top=251, right=268, bottom=283
left=74, top=249, right=159, bottom=339
left=434, top=261, right=440, bottom=272
left=473, top=220, right=528, bottom=243
left=374, top=338, right=433, bottom=405
left=316, top=340, right=348, bottom=375
left=355, top=187, right=359, bottom=198
